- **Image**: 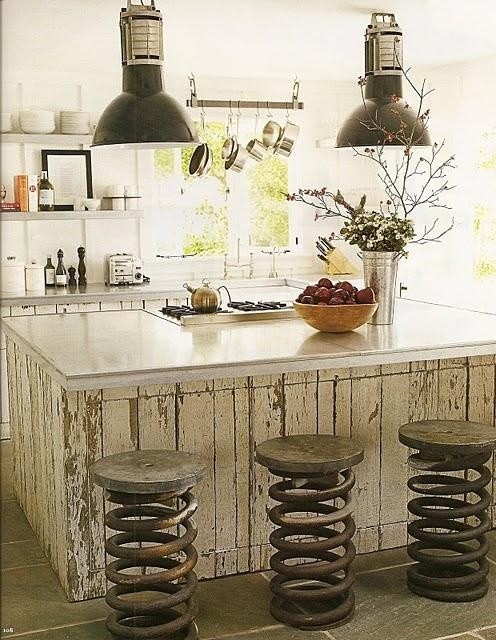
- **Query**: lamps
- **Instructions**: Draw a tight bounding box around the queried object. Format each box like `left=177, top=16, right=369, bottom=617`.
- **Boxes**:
left=90, top=0, right=198, bottom=151
left=335, top=12, right=433, bottom=148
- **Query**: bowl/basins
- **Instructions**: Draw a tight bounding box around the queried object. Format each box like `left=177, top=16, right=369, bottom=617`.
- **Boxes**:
left=85, top=199, right=101, bottom=211
left=292, top=298, right=379, bottom=333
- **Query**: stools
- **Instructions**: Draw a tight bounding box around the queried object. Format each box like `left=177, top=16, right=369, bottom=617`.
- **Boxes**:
left=90, top=448, right=207, bottom=639
left=398, top=421, right=496, bottom=602
left=255, top=434, right=366, bottom=630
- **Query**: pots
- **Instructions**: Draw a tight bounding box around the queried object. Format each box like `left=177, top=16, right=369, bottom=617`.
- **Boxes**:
left=221, top=125, right=249, bottom=173
left=262, top=120, right=282, bottom=149
left=188, top=116, right=213, bottom=179
left=273, top=121, right=300, bottom=158
left=246, top=139, right=267, bottom=162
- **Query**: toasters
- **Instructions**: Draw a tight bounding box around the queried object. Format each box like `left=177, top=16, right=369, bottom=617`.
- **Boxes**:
left=106, top=253, right=144, bottom=285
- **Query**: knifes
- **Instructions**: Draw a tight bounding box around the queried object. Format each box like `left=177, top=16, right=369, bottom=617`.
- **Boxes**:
left=316, top=235, right=334, bottom=264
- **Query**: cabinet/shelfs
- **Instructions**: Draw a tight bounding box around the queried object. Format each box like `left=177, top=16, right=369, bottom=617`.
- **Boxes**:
left=1, top=128, right=187, bottom=306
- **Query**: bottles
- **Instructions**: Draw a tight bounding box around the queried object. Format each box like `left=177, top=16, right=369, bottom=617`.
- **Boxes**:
left=44, top=255, right=55, bottom=287
left=37, top=170, right=55, bottom=211
left=1, top=257, right=26, bottom=293
left=26, top=260, right=46, bottom=291
left=55, top=249, right=68, bottom=288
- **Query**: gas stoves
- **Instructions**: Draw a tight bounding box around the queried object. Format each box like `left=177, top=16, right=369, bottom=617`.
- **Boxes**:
left=163, top=300, right=287, bottom=322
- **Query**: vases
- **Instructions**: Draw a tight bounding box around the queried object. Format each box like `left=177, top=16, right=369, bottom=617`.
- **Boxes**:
left=357, top=251, right=403, bottom=325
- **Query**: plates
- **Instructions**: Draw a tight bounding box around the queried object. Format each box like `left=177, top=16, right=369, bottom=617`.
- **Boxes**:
left=19, top=110, right=92, bottom=135
left=0, top=112, right=16, bottom=133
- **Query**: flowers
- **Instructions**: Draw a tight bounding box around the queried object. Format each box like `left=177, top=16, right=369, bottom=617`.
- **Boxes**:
left=280, top=185, right=416, bottom=251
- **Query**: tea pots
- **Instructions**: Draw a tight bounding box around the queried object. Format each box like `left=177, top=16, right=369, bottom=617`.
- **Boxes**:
left=183, top=278, right=233, bottom=313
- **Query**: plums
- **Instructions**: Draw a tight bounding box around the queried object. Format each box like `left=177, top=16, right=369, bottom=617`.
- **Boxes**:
left=298, top=278, right=375, bottom=306
